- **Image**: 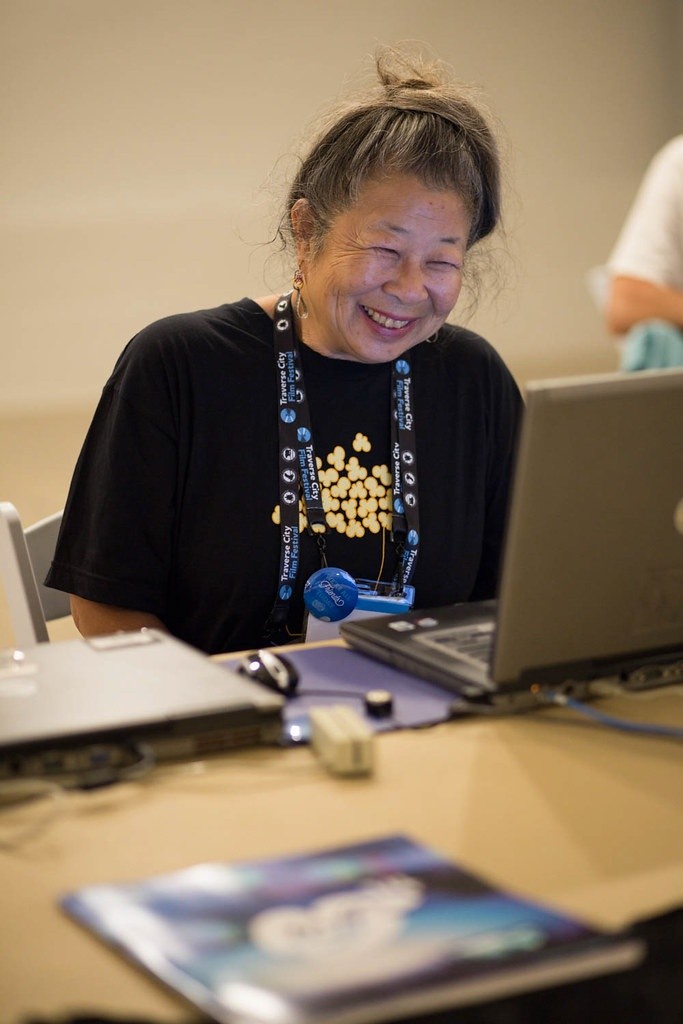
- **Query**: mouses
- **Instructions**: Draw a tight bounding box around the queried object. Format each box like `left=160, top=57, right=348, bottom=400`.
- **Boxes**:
left=237, top=649, right=299, bottom=697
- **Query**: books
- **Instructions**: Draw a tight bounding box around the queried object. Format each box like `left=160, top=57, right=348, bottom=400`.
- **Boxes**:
left=62, top=834, right=641, bottom=1024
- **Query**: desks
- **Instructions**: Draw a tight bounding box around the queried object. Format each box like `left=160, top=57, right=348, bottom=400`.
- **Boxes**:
left=1, top=636, right=683, bottom=1024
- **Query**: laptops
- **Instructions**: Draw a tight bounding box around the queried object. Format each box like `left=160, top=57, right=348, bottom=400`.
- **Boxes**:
left=339, top=367, right=683, bottom=705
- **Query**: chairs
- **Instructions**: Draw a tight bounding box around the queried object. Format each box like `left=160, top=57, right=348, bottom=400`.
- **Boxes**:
left=0, top=500, right=72, bottom=646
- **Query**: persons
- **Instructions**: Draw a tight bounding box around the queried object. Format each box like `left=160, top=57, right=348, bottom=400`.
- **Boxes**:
left=43, top=46, right=525, bottom=656
left=598, top=136, right=683, bottom=372
